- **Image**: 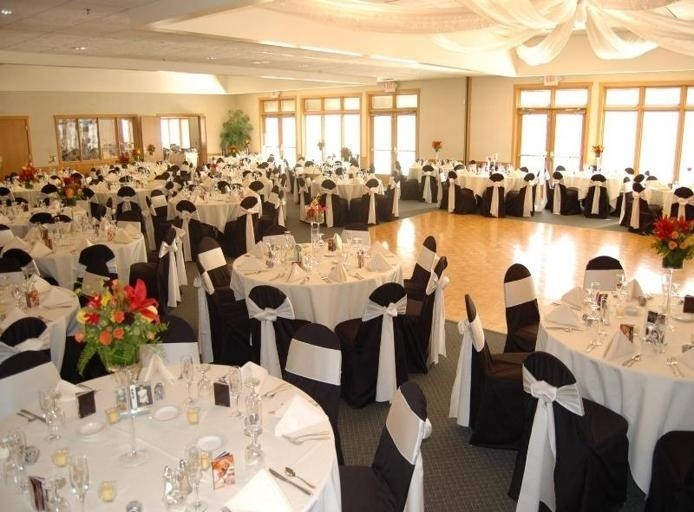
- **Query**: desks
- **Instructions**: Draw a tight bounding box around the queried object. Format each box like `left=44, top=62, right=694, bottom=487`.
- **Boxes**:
left=230, top=236, right=403, bottom=348
left=1, top=280, right=80, bottom=347
left=541, top=282, right=694, bottom=493
left=3, top=358, right=341, bottom=511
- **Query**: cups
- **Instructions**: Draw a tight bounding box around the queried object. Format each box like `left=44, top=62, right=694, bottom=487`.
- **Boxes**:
left=265, top=231, right=363, bottom=275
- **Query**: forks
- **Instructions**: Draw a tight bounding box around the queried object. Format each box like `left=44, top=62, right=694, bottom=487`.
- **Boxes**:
left=282, top=431, right=330, bottom=444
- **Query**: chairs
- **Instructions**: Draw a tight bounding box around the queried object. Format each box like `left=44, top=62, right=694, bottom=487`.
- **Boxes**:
left=459, top=293, right=535, bottom=449
left=192, top=236, right=246, bottom=347
left=242, top=284, right=296, bottom=376
left=340, top=381, right=432, bottom=512
left=393, top=257, right=448, bottom=375
left=0, top=316, right=52, bottom=359
left=0, top=140, right=404, bottom=269
left=170, top=216, right=189, bottom=289
left=671, top=187, right=693, bottom=226
left=642, top=429, right=694, bottom=511
left=283, top=324, right=345, bottom=466
left=0, top=247, right=60, bottom=287
left=130, top=225, right=186, bottom=309
left=412, top=155, right=668, bottom=236
left=144, top=311, right=202, bottom=371
left=73, top=246, right=118, bottom=306
left=501, top=263, right=540, bottom=355
left=405, top=234, right=438, bottom=299
left=1, top=352, right=58, bottom=412
left=347, top=281, right=410, bottom=409
left=582, top=254, right=624, bottom=295
left=514, top=352, right=631, bottom=512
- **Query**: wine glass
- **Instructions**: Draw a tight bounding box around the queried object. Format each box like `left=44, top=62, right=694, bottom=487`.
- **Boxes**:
left=10, top=281, right=25, bottom=312
left=584, top=272, right=680, bottom=353
left=0, top=355, right=264, bottom=512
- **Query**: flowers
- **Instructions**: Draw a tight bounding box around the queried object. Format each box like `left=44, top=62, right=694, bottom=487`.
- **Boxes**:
left=653, top=213, right=694, bottom=273
left=69, top=277, right=170, bottom=386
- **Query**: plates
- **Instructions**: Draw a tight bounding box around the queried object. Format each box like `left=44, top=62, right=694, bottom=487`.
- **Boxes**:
left=674, top=312, right=693, bottom=322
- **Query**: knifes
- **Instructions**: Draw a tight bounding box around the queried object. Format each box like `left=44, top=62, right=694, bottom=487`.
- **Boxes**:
left=545, top=325, right=584, bottom=332
left=268, top=469, right=310, bottom=496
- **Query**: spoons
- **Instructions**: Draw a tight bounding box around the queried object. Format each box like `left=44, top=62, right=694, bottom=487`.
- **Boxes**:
left=665, top=357, right=684, bottom=378
left=261, top=382, right=290, bottom=398
left=284, top=466, right=315, bottom=489
left=621, top=353, right=641, bottom=368
